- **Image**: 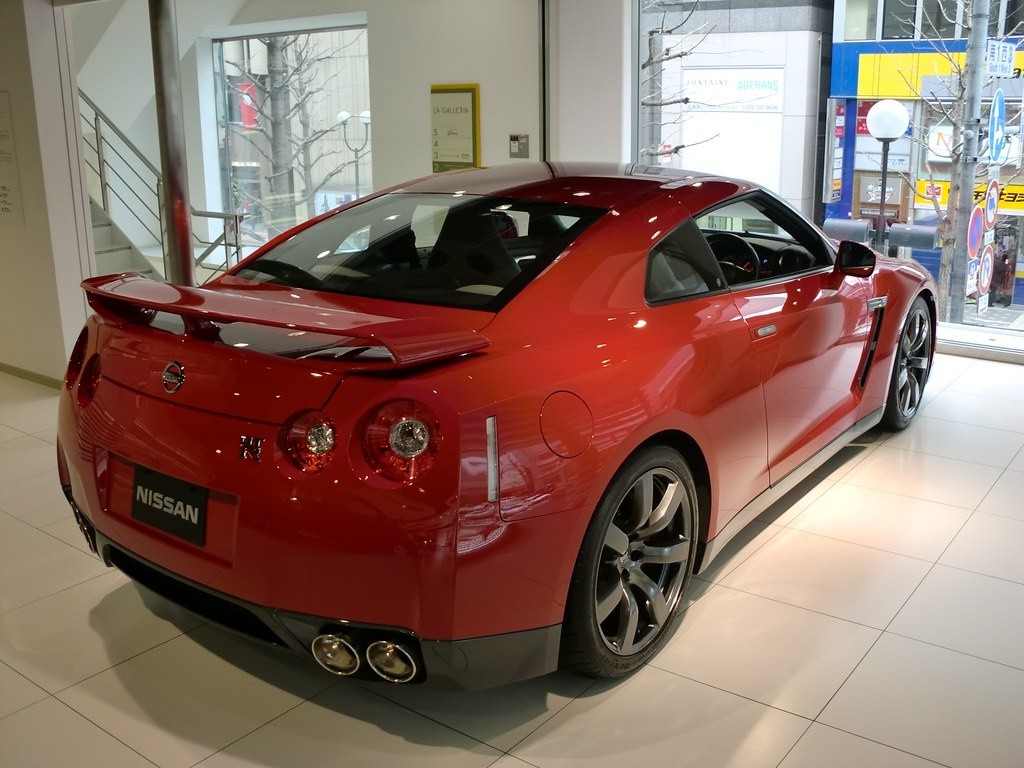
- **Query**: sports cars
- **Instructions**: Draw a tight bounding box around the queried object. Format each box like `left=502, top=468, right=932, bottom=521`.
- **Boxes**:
left=51, top=158, right=941, bottom=690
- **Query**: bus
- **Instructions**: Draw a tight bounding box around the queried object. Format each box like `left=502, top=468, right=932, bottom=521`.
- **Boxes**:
left=232, top=162, right=260, bottom=214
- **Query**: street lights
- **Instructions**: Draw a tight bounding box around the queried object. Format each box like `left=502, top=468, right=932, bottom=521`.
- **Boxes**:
left=821, top=100, right=940, bottom=262
left=338, top=111, right=371, bottom=201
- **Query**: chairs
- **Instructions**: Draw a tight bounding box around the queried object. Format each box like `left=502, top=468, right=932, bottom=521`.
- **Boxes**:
left=428, top=210, right=522, bottom=290
